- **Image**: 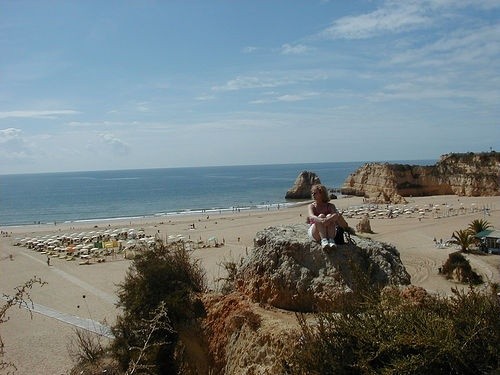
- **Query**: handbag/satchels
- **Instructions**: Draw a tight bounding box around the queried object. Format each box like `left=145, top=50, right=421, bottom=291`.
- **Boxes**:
left=334, top=226, right=345, bottom=244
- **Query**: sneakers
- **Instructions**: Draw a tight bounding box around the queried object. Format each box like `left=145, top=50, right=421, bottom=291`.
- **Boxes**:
left=329, top=238, right=337, bottom=248
left=321, top=238, right=328, bottom=250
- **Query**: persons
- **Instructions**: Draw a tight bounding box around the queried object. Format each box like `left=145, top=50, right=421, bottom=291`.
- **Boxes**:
left=307, top=184, right=340, bottom=250
